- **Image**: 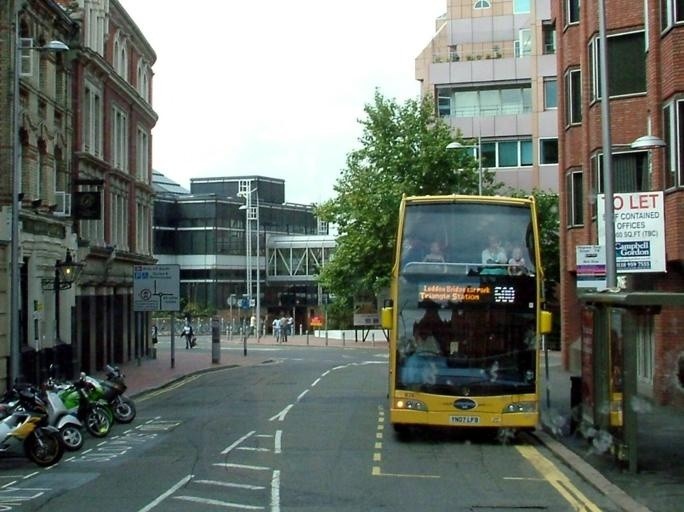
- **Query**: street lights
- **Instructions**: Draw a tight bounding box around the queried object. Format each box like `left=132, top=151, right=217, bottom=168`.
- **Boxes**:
left=11, top=14, right=71, bottom=378
left=238, top=205, right=261, bottom=343
left=444, top=140, right=483, bottom=195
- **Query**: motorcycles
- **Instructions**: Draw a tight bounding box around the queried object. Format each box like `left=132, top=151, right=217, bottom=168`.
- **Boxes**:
left=0, top=363, right=136, bottom=467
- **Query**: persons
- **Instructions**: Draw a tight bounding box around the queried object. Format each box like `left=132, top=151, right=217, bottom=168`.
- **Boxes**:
left=151, top=324, right=158, bottom=347
left=272, top=315, right=280, bottom=340
left=404, top=233, right=427, bottom=263
left=286, top=313, right=294, bottom=336
left=179, top=320, right=194, bottom=349
left=481, top=235, right=505, bottom=268
left=422, top=240, right=450, bottom=274
left=404, top=320, right=447, bottom=362
left=278, top=311, right=288, bottom=342
left=508, top=248, right=527, bottom=278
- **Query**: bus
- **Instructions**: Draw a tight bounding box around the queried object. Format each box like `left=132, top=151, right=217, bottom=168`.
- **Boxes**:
left=379, top=193, right=554, bottom=431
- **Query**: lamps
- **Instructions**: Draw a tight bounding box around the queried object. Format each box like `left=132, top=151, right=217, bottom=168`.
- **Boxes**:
left=43, top=249, right=86, bottom=290
